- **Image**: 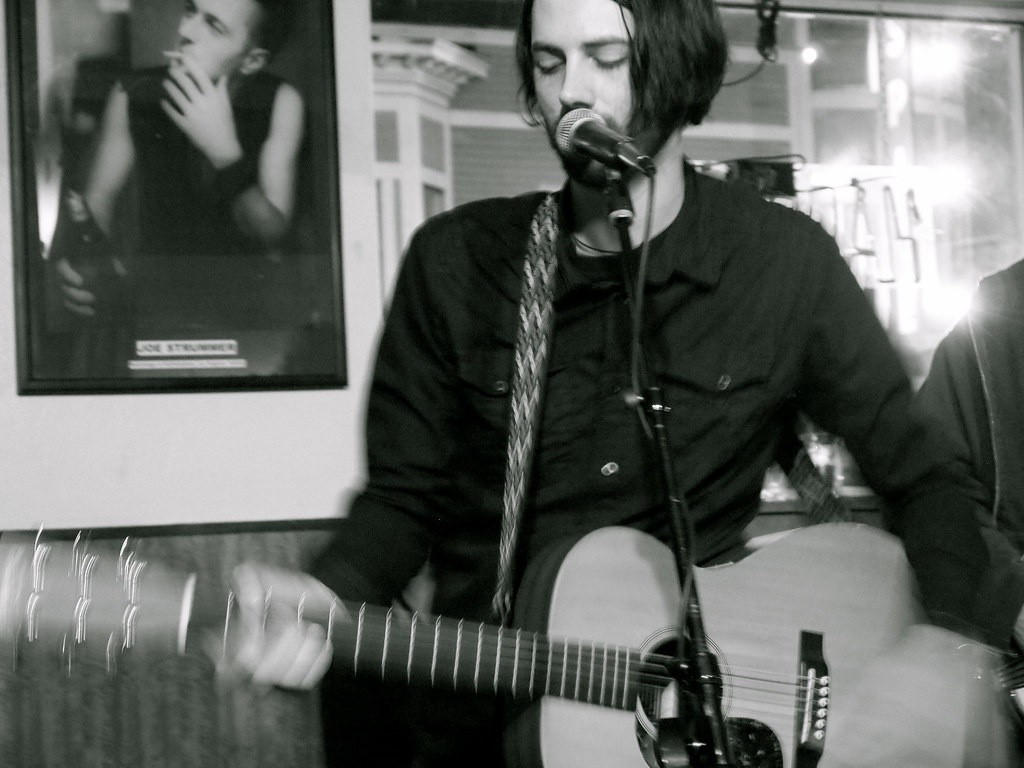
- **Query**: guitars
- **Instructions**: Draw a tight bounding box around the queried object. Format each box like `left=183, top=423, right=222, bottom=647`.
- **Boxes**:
left=173, top=502, right=1024, bottom=768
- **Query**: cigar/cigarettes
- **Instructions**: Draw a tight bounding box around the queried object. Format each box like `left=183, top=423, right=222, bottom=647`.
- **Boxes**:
left=162, top=49, right=184, bottom=59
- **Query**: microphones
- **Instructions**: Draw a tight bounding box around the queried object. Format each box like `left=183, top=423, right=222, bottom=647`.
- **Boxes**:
left=555, top=109, right=657, bottom=178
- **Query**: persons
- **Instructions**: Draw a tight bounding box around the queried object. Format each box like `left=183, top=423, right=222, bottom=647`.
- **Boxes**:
left=916, top=246, right=1023, bottom=643
left=213, top=0, right=1023, bottom=766
left=51, top=0, right=307, bottom=382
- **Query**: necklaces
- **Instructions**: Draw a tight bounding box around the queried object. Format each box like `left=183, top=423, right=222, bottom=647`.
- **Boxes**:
left=571, top=233, right=624, bottom=255
